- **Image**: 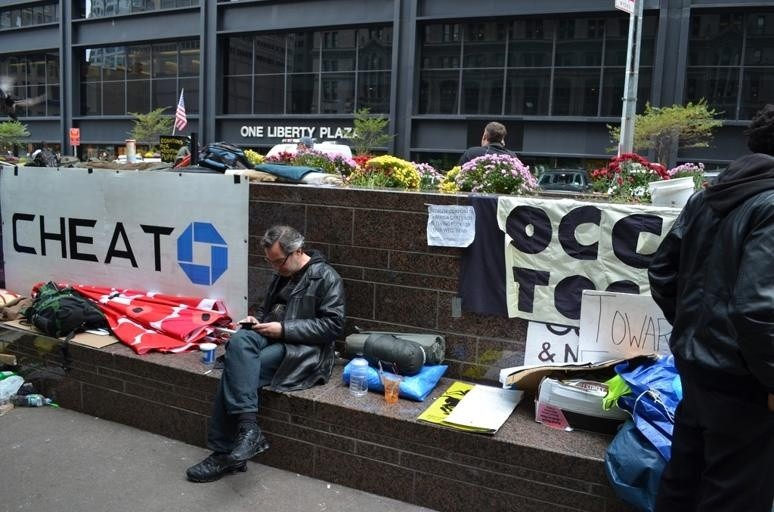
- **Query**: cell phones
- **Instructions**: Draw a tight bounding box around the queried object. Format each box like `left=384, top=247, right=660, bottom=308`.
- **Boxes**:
left=240, top=322, right=257, bottom=330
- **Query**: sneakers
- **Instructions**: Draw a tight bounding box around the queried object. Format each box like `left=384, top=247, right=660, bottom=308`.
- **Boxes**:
left=184, top=449, right=250, bottom=484
left=225, top=423, right=271, bottom=463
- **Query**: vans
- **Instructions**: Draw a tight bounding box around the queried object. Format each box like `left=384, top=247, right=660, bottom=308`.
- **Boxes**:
left=535, top=167, right=595, bottom=192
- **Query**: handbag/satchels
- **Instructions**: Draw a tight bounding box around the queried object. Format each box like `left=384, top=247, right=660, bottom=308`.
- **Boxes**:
left=600, top=350, right=686, bottom=464
left=342, top=356, right=450, bottom=402
left=362, top=331, right=427, bottom=374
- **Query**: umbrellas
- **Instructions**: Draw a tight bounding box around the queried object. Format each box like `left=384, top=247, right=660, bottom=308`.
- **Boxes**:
left=29, top=281, right=238, bottom=355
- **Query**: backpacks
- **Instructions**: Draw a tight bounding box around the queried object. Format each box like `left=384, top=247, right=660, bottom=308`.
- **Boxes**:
left=19, top=285, right=112, bottom=335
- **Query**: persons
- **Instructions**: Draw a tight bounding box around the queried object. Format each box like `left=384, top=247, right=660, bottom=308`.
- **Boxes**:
left=644, top=103, right=773, bottom=512
left=455, top=120, right=518, bottom=169
left=185, top=223, right=344, bottom=486
left=293, top=137, right=313, bottom=153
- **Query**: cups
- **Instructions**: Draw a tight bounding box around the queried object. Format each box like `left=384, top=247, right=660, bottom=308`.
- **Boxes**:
left=199, top=342, right=217, bottom=364
left=125, top=139, right=136, bottom=163
left=383, top=375, right=400, bottom=402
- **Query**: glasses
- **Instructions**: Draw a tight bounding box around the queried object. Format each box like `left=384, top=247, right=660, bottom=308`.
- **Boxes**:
left=263, top=253, right=293, bottom=268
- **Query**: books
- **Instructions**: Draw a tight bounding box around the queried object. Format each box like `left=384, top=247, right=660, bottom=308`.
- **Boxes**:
left=443, top=384, right=524, bottom=431
left=416, top=381, right=525, bottom=435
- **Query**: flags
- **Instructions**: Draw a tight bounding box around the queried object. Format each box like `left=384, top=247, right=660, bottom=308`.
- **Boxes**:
left=174, top=92, right=187, bottom=132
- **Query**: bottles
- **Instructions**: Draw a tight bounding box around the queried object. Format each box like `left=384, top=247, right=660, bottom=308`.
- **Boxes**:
left=349, top=352, right=369, bottom=398
left=13, top=394, right=53, bottom=407
